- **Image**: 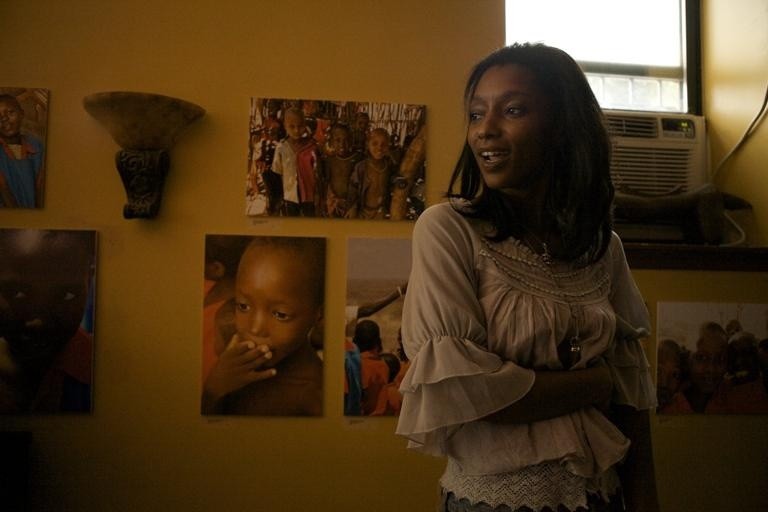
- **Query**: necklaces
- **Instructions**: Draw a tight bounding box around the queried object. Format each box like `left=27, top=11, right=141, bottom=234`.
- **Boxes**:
left=520, top=210, right=562, bottom=272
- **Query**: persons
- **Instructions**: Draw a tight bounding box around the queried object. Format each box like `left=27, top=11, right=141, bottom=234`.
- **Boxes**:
left=658, top=313, right=767, bottom=414
left=389, top=43, right=663, bottom=511
left=0, top=230, right=95, bottom=414
left=344, top=278, right=411, bottom=415
left=0, top=94, right=43, bottom=208
left=201, top=236, right=326, bottom=415
left=247, top=95, right=428, bottom=222
left=206, top=236, right=242, bottom=313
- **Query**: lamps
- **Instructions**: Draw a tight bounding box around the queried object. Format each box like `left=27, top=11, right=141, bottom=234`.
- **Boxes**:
left=81, top=91, right=206, bottom=219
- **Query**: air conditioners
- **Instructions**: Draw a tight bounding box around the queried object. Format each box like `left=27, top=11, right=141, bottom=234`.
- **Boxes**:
left=602, top=112, right=710, bottom=241
